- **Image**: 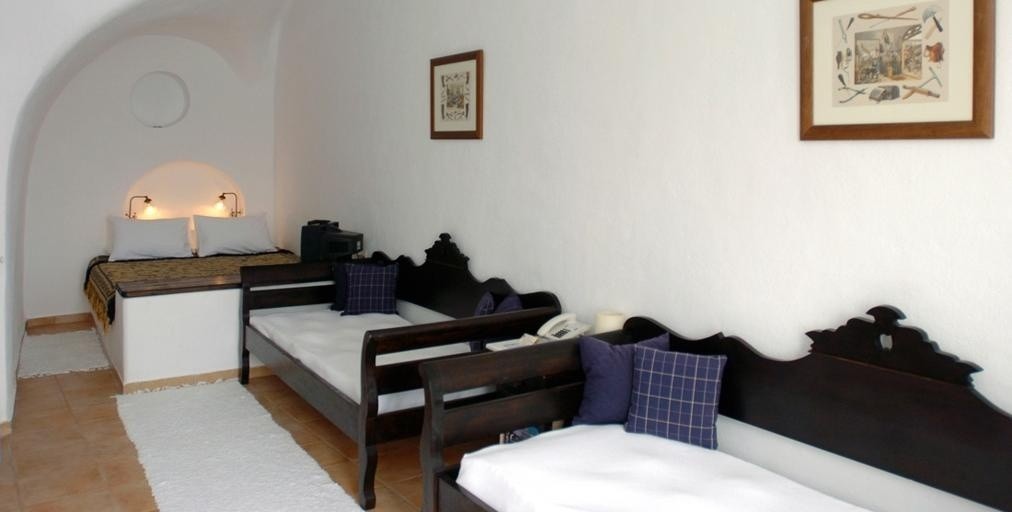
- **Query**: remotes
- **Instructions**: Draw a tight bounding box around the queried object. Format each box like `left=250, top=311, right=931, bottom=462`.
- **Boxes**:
left=307, top=219, right=330, bottom=225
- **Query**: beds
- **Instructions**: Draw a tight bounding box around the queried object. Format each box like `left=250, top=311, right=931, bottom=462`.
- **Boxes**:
left=74, top=250, right=312, bottom=388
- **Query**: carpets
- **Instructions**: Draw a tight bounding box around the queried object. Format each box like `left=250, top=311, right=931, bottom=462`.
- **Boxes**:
left=105, top=373, right=371, bottom=512
left=17, top=322, right=110, bottom=381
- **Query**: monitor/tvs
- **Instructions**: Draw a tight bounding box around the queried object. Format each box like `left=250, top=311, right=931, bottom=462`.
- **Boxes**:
left=302, top=221, right=363, bottom=264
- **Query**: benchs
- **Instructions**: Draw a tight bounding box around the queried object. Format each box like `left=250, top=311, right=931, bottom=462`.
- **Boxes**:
left=412, top=304, right=1012, bottom=512
left=236, top=235, right=562, bottom=512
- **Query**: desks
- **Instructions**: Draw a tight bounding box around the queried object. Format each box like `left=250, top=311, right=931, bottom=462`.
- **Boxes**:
left=483, top=338, right=564, bottom=433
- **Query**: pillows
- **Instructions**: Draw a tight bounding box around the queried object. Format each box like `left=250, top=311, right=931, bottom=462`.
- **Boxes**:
left=571, top=330, right=678, bottom=428
left=624, top=343, right=729, bottom=452
left=193, top=210, right=278, bottom=258
left=108, top=214, right=193, bottom=263
left=327, top=261, right=403, bottom=319
left=465, top=289, right=526, bottom=354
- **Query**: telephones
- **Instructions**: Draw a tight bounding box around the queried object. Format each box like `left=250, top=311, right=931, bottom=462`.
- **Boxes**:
left=537, top=312, right=591, bottom=341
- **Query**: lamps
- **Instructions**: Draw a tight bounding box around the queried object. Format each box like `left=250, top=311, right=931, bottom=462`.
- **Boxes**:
left=127, top=193, right=158, bottom=221
left=593, top=308, right=626, bottom=336
left=213, top=191, right=242, bottom=218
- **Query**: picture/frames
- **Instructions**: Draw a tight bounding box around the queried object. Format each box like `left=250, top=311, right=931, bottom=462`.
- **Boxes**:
left=427, top=49, right=485, bottom=141
left=794, top=0, right=998, bottom=145
